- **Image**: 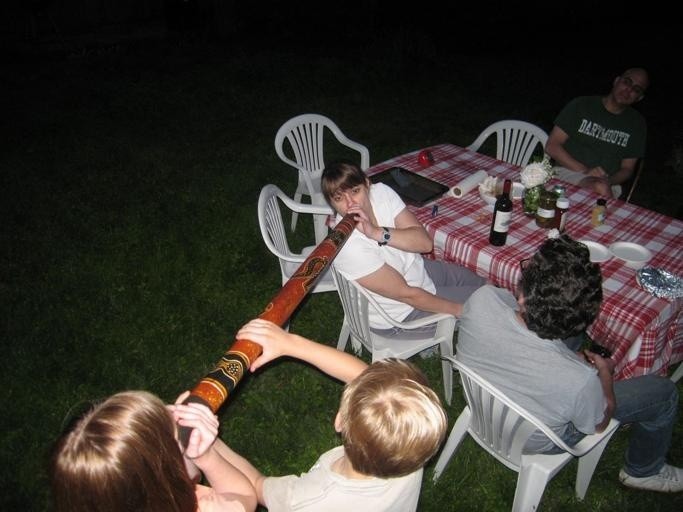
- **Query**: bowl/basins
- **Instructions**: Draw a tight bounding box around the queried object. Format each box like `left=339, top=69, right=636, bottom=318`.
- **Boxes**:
left=479, top=189, right=497, bottom=206
left=512, top=182, right=525, bottom=200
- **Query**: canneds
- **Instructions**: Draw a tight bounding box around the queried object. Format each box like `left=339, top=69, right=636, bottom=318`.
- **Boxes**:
left=535, top=190, right=557, bottom=230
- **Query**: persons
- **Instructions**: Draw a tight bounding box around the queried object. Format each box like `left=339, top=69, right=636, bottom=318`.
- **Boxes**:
left=543, top=65, right=650, bottom=202
left=455, top=232, right=683, bottom=494
left=319, top=160, right=488, bottom=342
left=174, top=318, right=450, bottom=512
left=48, top=387, right=259, bottom=512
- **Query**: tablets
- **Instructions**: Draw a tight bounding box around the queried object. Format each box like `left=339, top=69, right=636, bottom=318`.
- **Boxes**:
left=368, top=165, right=449, bottom=207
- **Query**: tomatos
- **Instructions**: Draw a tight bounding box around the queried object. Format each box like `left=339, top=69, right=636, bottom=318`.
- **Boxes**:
left=417, top=149, right=435, bottom=168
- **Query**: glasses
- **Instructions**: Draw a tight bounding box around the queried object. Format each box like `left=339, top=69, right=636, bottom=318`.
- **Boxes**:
left=621, top=75, right=646, bottom=96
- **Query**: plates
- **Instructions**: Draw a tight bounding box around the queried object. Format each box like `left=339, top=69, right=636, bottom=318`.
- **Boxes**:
left=577, top=240, right=610, bottom=263
left=608, top=242, right=652, bottom=264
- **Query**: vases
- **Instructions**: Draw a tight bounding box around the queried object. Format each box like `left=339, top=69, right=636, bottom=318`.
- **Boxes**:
left=521, top=185, right=546, bottom=220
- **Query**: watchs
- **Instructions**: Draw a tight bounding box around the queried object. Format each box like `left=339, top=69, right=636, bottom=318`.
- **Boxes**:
left=377, top=225, right=391, bottom=247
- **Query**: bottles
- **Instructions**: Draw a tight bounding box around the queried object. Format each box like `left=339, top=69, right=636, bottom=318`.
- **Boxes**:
left=488, top=178, right=514, bottom=247
left=534, top=182, right=571, bottom=232
left=592, top=198, right=607, bottom=225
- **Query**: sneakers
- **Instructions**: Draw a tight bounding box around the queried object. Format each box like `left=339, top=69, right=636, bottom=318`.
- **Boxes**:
left=618, top=462, right=683, bottom=494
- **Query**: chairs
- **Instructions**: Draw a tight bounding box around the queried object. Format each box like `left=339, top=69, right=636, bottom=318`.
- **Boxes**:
left=615, top=118, right=648, bottom=203
left=257, top=184, right=363, bottom=358
left=670, top=361, right=683, bottom=383
left=329, top=260, right=457, bottom=407
left=274, top=112, right=370, bottom=246
left=431, top=352, right=621, bottom=512
left=466, top=119, right=552, bottom=169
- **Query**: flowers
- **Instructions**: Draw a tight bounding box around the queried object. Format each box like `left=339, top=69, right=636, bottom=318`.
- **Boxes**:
left=519, top=158, right=552, bottom=212
